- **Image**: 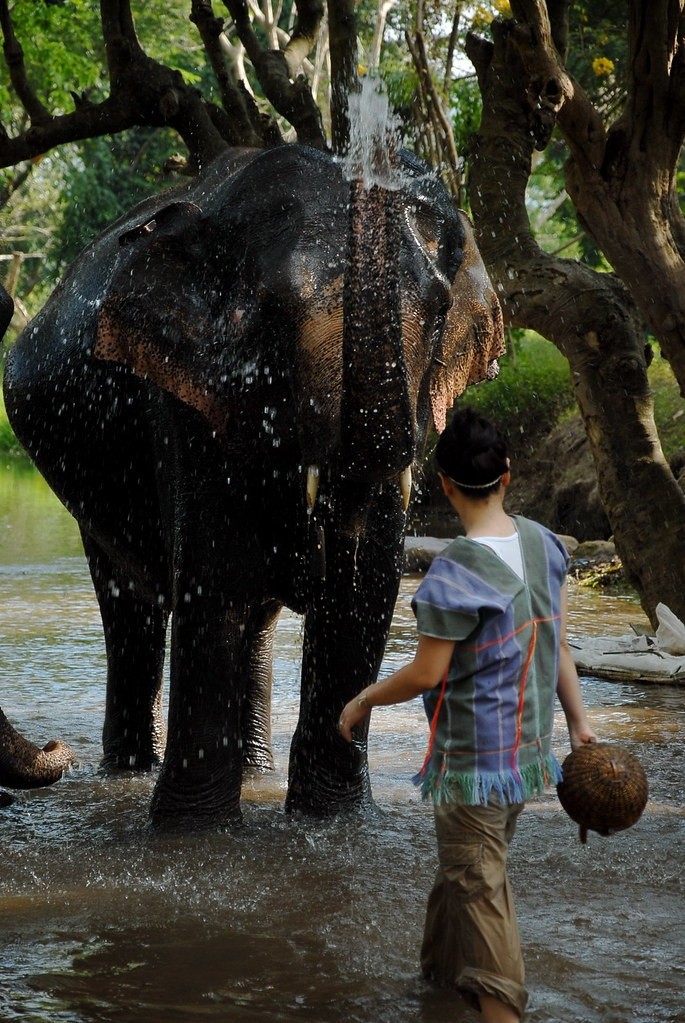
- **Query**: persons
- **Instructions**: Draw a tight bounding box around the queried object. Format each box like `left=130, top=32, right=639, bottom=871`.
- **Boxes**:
left=339, top=409, right=599, bottom=1023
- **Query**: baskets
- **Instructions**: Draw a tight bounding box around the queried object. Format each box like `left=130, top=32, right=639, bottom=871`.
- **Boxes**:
left=556, top=741, right=649, bottom=844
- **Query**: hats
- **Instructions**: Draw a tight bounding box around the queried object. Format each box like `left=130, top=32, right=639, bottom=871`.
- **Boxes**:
left=433, top=413, right=513, bottom=490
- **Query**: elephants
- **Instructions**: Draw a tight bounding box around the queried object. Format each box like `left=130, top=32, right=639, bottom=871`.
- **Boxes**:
left=1, top=129, right=504, bottom=843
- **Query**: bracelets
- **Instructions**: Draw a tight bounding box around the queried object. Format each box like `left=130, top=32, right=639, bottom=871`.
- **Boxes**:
left=358, top=694, right=370, bottom=709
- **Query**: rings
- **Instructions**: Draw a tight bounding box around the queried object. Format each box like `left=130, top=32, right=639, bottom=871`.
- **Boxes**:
left=339, top=721, right=343, bottom=726
